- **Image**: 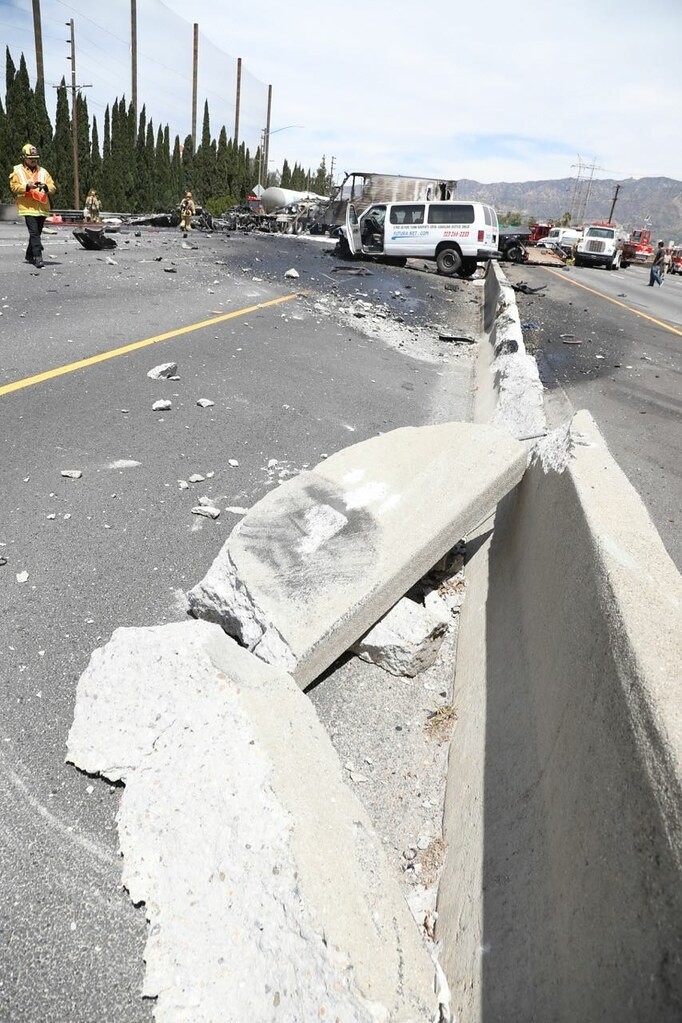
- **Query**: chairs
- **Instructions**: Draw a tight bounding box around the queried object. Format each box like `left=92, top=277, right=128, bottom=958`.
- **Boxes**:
left=404, top=214, right=413, bottom=224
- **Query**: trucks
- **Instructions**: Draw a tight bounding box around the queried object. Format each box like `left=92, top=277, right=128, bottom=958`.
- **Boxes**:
left=225, top=171, right=455, bottom=237
left=538, top=227, right=579, bottom=254
left=498, top=225, right=549, bottom=246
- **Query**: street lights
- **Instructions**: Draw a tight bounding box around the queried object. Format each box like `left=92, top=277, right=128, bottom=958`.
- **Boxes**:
left=259, top=124, right=303, bottom=197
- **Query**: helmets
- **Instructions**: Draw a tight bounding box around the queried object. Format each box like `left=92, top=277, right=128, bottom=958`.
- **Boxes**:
left=186, top=192, right=193, bottom=197
left=20, top=144, right=40, bottom=159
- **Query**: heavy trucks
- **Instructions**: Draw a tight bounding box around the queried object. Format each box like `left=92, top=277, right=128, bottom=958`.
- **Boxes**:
left=575, top=222, right=625, bottom=269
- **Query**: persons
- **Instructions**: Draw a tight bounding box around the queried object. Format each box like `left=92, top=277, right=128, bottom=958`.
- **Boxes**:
left=647, top=242, right=672, bottom=288
left=9, top=143, right=56, bottom=267
left=178, top=191, right=196, bottom=231
left=83, top=191, right=102, bottom=224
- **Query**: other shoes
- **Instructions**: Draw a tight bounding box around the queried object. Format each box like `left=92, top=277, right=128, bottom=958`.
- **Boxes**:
left=645, top=284, right=654, bottom=287
left=659, top=281, right=665, bottom=287
left=25, top=252, right=45, bottom=268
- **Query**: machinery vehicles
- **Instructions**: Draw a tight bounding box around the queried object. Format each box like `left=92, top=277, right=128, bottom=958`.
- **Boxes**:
left=622, top=226, right=651, bottom=268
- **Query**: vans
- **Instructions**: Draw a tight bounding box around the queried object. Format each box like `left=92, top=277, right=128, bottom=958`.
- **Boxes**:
left=336, top=199, right=503, bottom=278
left=667, top=247, right=682, bottom=276
